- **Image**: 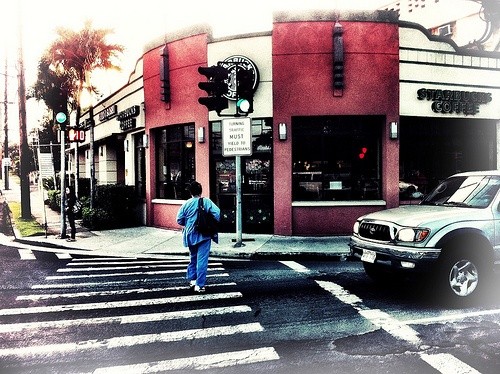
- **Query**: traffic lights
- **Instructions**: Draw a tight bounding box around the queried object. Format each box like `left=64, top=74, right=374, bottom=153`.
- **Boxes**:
left=196, top=64, right=227, bottom=111
left=237, top=69, right=255, bottom=114
left=54, top=108, right=69, bottom=130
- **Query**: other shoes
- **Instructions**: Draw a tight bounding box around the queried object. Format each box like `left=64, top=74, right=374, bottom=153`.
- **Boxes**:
left=189, top=280, right=197, bottom=287
left=194, top=285, right=205, bottom=292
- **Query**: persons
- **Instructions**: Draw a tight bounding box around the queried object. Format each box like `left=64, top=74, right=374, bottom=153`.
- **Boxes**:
left=177, top=182, right=220, bottom=292
left=65, top=186, right=76, bottom=242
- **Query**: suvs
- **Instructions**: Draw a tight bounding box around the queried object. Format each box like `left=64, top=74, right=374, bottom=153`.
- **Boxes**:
left=347, top=170, right=500, bottom=308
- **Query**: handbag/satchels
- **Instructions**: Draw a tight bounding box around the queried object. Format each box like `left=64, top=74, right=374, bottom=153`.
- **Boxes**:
left=195, top=198, right=218, bottom=235
